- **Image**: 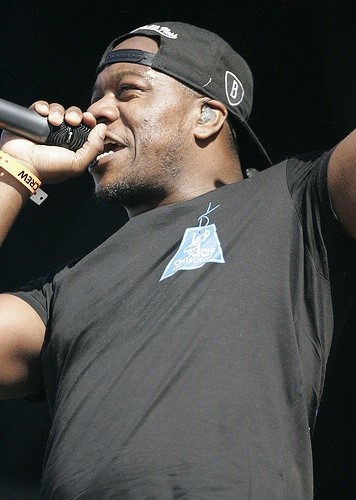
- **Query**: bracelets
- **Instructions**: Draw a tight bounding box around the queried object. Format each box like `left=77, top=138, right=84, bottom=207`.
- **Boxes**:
left=0, top=150, right=49, bottom=205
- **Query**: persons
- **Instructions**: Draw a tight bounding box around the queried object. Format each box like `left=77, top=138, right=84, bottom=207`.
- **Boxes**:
left=0, top=22, right=356, bottom=500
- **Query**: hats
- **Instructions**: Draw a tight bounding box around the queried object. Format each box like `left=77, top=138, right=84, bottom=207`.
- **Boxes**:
left=94, top=22, right=273, bottom=178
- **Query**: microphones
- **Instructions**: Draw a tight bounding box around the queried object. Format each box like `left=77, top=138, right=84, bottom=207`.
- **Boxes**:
left=0, top=98, right=91, bottom=152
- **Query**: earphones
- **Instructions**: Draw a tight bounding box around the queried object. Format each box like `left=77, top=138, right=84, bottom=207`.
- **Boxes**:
left=198, top=107, right=215, bottom=124
